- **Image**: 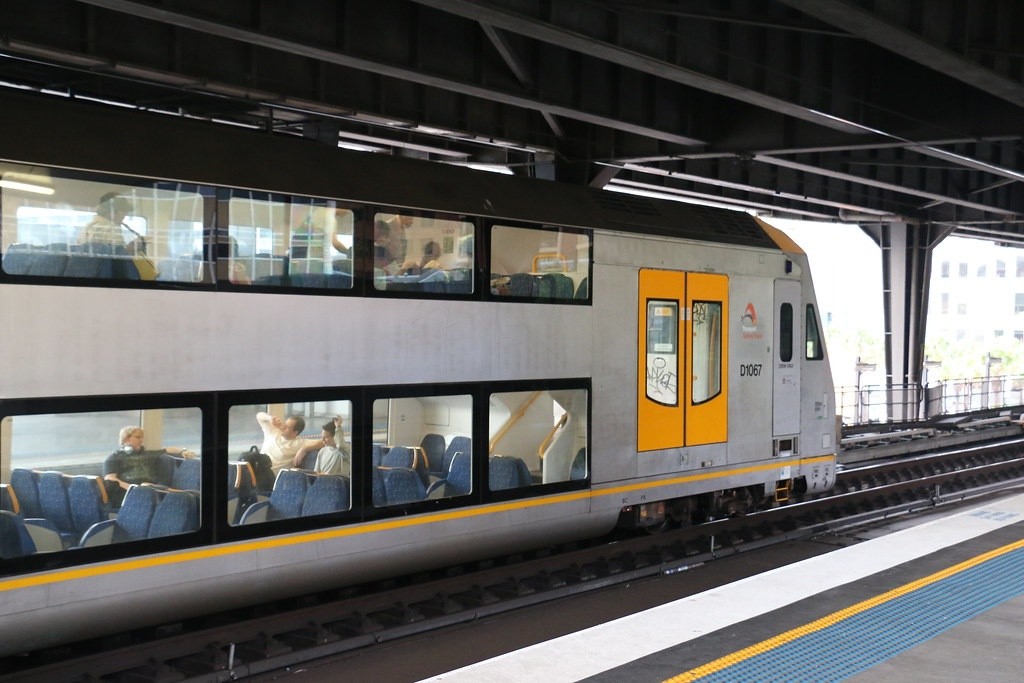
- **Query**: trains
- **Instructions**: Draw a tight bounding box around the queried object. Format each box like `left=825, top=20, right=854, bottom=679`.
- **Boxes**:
left=0, top=80, right=848, bottom=668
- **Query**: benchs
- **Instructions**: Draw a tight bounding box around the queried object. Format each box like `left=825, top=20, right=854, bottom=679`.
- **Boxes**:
left=1, top=243, right=588, bottom=299
left=0, top=433, right=535, bottom=560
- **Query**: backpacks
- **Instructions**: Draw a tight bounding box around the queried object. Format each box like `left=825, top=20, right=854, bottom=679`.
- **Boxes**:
left=238, top=445, right=275, bottom=496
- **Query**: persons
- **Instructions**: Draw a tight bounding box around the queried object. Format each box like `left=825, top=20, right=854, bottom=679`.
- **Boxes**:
left=284, top=207, right=347, bottom=260
left=104, top=425, right=196, bottom=509
left=373, top=215, right=413, bottom=290
left=311, top=414, right=350, bottom=479
left=82, top=190, right=155, bottom=255
left=256, top=412, right=325, bottom=480
left=196, top=233, right=252, bottom=285
left=387, top=241, right=442, bottom=277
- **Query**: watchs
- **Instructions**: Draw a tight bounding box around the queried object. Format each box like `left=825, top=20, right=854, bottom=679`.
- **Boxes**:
left=179, top=448, right=188, bottom=455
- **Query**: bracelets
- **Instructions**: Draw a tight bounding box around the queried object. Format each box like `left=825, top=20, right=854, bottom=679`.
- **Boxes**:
left=335, top=425, right=342, bottom=427
